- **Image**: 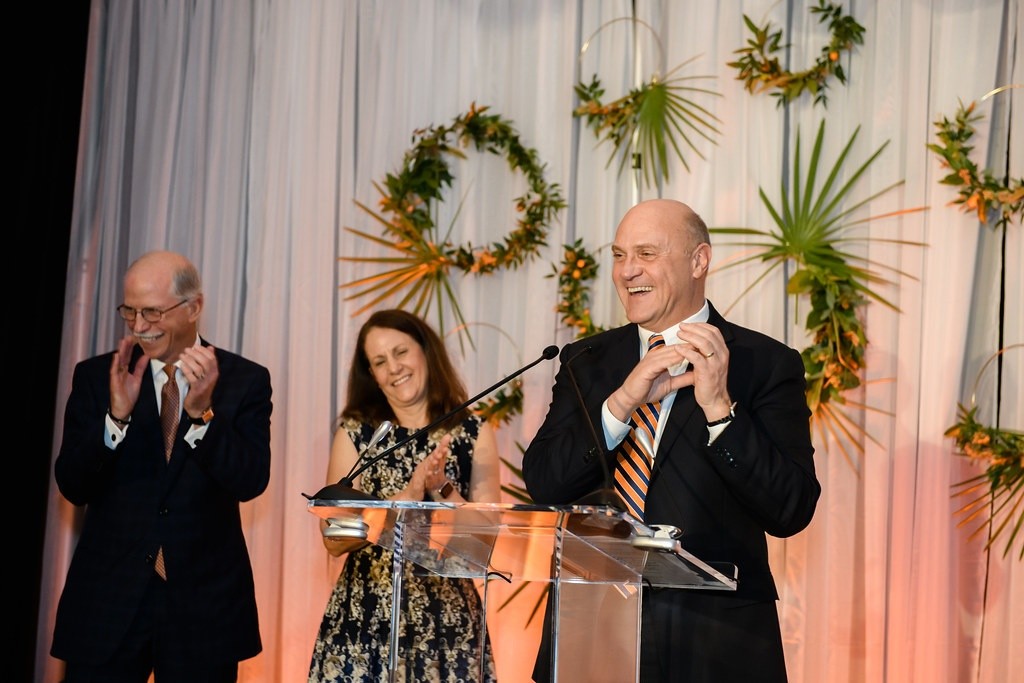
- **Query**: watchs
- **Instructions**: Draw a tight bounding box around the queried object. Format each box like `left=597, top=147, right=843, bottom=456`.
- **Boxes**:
left=707, top=402, right=738, bottom=427
left=431, top=478, right=454, bottom=501
left=186, top=405, right=214, bottom=426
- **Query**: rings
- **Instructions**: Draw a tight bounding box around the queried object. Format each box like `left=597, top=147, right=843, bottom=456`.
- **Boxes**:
left=198, top=371, right=206, bottom=380
left=433, top=467, right=442, bottom=474
left=118, top=365, right=125, bottom=371
left=704, top=351, right=715, bottom=358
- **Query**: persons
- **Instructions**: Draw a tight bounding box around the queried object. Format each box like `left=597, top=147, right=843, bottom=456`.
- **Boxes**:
left=49, top=249, right=273, bottom=683
left=521, top=199, right=823, bottom=682
left=304, top=308, right=503, bottom=683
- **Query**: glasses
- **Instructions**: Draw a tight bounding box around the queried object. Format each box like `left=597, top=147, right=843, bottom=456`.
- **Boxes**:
left=117, top=299, right=187, bottom=323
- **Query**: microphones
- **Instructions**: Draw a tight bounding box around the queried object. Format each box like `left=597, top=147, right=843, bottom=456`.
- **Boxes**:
left=565, top=340, right=629, bottom=512
left=311, top=345, right=558, bottom=501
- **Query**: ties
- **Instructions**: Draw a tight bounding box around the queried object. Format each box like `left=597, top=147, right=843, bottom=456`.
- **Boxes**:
left=154, top=365, right=180, bottom=582
left=611, top=334, right=668, bottom=599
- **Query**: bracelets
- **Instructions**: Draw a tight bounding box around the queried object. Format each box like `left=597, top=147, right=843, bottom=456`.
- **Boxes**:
left=107, top=406, right=133, bottom=425
